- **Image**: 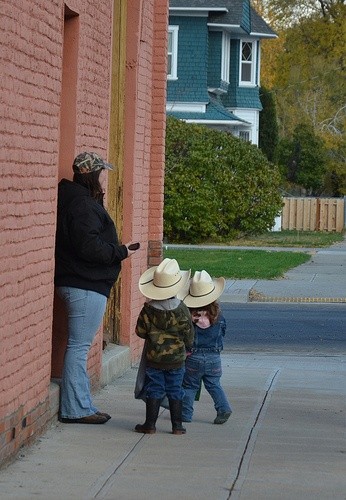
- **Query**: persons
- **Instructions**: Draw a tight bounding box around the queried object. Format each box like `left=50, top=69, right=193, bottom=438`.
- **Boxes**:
left=181, top=270, right=232, bottom=424
left=55, top=152, right=136, bottom=424
left=134, top=257, right=195, bottom=434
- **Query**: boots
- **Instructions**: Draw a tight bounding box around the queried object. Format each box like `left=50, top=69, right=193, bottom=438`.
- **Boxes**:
left=135, top=398, right=161, bottom=434
left=169, top=399, right=186, bottom=434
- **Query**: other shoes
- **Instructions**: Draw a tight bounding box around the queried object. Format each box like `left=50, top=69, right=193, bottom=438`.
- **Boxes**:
left=214, top=411, right=232, bottom=424
left=62, top=411, right=112, bottom=423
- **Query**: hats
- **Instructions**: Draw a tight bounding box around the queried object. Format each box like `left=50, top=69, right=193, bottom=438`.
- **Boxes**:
left=183, top=270, right=225, bottom=308
left=139, top=258, right=191, bottom=300
left=73, top=151, right=114, bottom=174
